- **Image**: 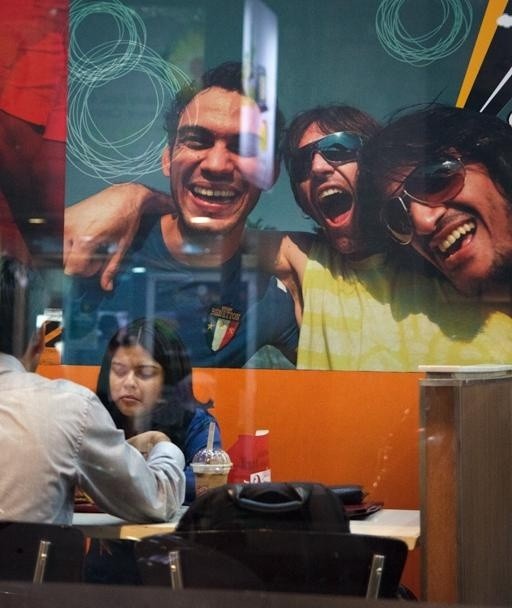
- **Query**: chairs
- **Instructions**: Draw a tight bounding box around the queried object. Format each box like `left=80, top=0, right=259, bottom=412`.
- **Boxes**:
left=133, top=530, right=407, bottom=598
left=0, top=519, right=86, bottom=584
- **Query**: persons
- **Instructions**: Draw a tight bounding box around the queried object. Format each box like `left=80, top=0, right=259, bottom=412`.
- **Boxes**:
left=96, top=316, right=222, bottom=506
left=62, top=59, right=299, bottom=368
left=354, top=87, right=512, bottom=298
left=63, top=100, right=512, bottom=372
left=0, top=252, right=187, bottom=581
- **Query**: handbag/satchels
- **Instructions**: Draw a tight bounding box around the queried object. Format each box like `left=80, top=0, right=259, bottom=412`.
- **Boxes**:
left=175, top=482, right=349, bottom=533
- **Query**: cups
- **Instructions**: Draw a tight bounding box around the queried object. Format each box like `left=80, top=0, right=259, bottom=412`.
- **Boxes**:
left=191, top=448, right=232, bottom=500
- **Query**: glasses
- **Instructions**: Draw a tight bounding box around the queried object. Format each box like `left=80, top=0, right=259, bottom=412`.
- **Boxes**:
left=376, top=150, right=465, bottom=245
left=286, top=131, right=363, bottom=185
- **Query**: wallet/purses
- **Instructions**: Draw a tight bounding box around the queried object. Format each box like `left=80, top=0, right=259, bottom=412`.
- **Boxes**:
left=325, top=485, right=383, bottom=520
